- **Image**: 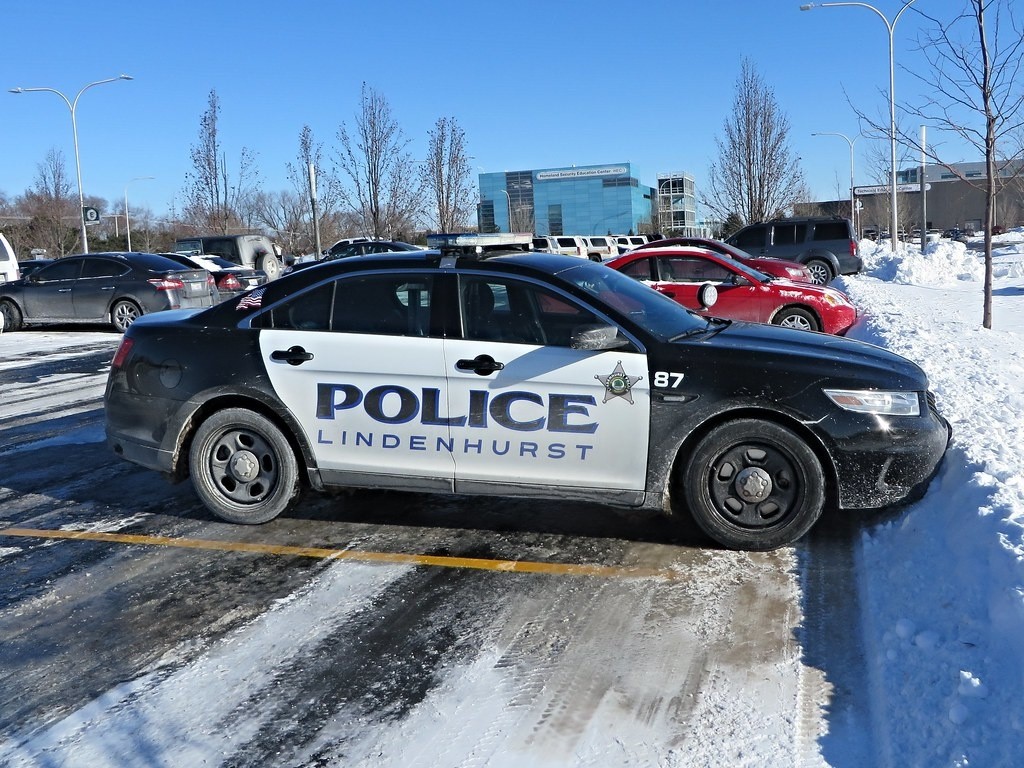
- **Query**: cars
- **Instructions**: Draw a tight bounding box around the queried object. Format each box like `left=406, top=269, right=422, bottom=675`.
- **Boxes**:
left=536, top=246, right=857, bottom=338
left=0, top=252, right=222, bottom=332
left=18, top=258, right=57, bottom=280
left=102, top=231, right=952, bottom=553
left=600, top=237, right=813, bottom=285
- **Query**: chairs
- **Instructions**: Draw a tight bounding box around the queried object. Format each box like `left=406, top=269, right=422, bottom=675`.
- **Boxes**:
left=463, top=282, right=504, bottom=341
left=506, top=290, right=554, bottom=344
left=661, top=262, right=680, bottom=281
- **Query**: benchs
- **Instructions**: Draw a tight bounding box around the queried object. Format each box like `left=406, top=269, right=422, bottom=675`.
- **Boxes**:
left=294, top=286, right=398, bottom=333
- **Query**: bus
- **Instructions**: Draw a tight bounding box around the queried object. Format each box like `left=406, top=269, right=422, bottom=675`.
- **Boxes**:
left=0, top=233, right=21, bottom=282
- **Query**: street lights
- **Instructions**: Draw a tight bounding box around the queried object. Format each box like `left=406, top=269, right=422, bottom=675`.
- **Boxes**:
left=499, top=189, right=513, bottom=233
left=124, top=177, right=155, bottom=253
left=7, top=73, right=136, bottom=254
left=799, top=0, right=916, bottom=253
left=810, top=128, right=880, bottom=231
left=659, top=179, right=683, bottom=233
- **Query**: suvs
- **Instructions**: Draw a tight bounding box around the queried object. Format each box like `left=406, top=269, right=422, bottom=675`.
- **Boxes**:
left=156, top=233, right=666, bottom=313
left=721, top=216, right=863, bottom=288
